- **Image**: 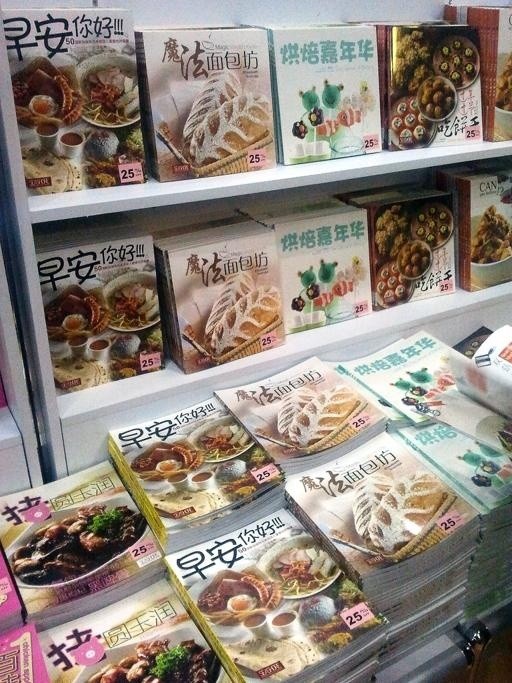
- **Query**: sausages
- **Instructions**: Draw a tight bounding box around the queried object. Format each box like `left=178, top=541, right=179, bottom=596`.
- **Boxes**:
left=267, top=580, right=282, bottom=610
left=242, top=576, right=270, bottom=604
left=172, top=447, right=193, bottom=467
left=189, top=452, right=203, bottom=470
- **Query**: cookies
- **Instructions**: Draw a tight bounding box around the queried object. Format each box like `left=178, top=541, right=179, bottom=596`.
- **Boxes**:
left=372, top=203, right=450, bottom=306
left=393, top=30, right=475, bottom=146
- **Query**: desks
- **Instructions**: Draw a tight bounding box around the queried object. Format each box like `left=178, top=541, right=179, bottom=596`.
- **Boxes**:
left=456, top=602, right=512, bottom=644
left=341, top=626, right=474, bottom=682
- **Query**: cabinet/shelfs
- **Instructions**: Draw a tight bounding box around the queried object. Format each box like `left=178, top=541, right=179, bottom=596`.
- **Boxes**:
left=2, top=247, right=45, bottom=499
left=2, top=0, right=510, bottom=482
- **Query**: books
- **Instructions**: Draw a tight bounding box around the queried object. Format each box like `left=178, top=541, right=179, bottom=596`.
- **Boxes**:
left=2, top=6, right=511, bottom=195
left=33, top=167, right=512, bottom=395
left=0, top=327, right=512, bottom=683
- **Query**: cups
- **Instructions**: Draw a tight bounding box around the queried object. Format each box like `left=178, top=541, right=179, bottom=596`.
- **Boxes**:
left=325, top=298, right=357, bottom=325
left=299, top=281, right=339, bottom=312
left=167, top=472, right=191, bottom=491
left=35, top=122, right=58, bottom=148
left=301, top=108, right=345, bottom=147
left=332, top=136, right=365, bottom=157
left=66, top=335, right=87, bottom=357
left=87, top=337, right=110, bottom=360
left=500, top=187, right=511, bottom=219
left=271, top=609, right=298, bottom=638
left=59, top=130, right=85, bottom=159
left=243, top=613, right=268, bottom=636
left=192, top=471, right=214, bottom=489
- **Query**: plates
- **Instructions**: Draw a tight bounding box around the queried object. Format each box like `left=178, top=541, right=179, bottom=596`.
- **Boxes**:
left=188, top=415, right=255, bottom=462
left=411, top=201, right=454, bottom=249
left=104, top=271, right=161, bottom=331
left=418, top=76, right=458, bottom=122
left=124, top=444, right=196, bottom=490
left=376, top=260, right=415, bottom=308
left=187, top=578, right=249, bottom=637
left=257, top=535, right=342, bottom=598
left=9, top=53, right=83, bottom=128
left=387, top=95, right=437, bottom=150
left=42, top=282, right=111, bottom=343
left=72, top=626, right=225, bottom=682
left=433, top=35, right=481, bottom=90
left=76, top=53, right=141, bottom=127
left=4, top=493, right=151, bottom=588
left=397, top=239, right=433, bottom=279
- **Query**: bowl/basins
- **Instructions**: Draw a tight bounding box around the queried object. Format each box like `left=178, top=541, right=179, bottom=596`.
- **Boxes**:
left=470, top=257, right=512, bottom=285
left=286, top=140, right=331, bottom=163
left=286, top=310, right=326, bottom=333
left=495, top=106, right=512, bottom=135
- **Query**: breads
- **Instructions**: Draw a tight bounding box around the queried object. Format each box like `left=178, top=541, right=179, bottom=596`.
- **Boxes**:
left=352, top=471, right=393, bottom=539
left=369, top=470, right=445, bottom=556
left=209, top=285, right=283, bottom=356
left=182, top=67, right=242, bottom=144
left=189, top=92, right=269, bottom=165
left=276, top=385, right=318, bottom=436
left=205, top=272, right=254, bottom=341
left=288, top=385, right=359, bottom=445
